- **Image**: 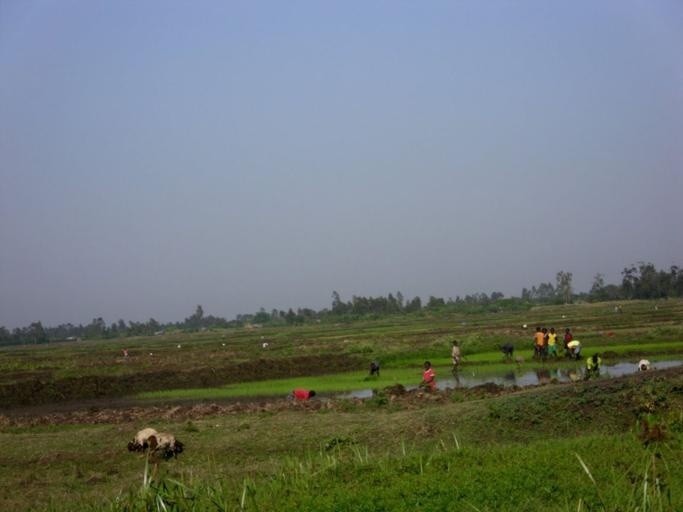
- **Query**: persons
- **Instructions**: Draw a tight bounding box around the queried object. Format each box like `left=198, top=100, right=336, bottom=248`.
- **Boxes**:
left=502, top=343, right=513, bottom=360
left=585, top=353, right=602, bottom=380
left=614, top=303, right=623, bottom=313
left=531, top=327, right=557, bottom=361
left=287, top=389, right=316, bottom=401
left=564, top=328, right=572, bottom=358
left=564, top=339, right=581, bottom=361
left=369, top=359, right=379, bottom=376
left=419, top=360, right=436, bottom=392
left=451, top=340, right=461, bottom=371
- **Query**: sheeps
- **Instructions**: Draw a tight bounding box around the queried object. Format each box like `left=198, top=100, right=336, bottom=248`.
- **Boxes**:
left=496, top=342, right=514, bottom=358
left=127, top=426, right=184, bottom=461
